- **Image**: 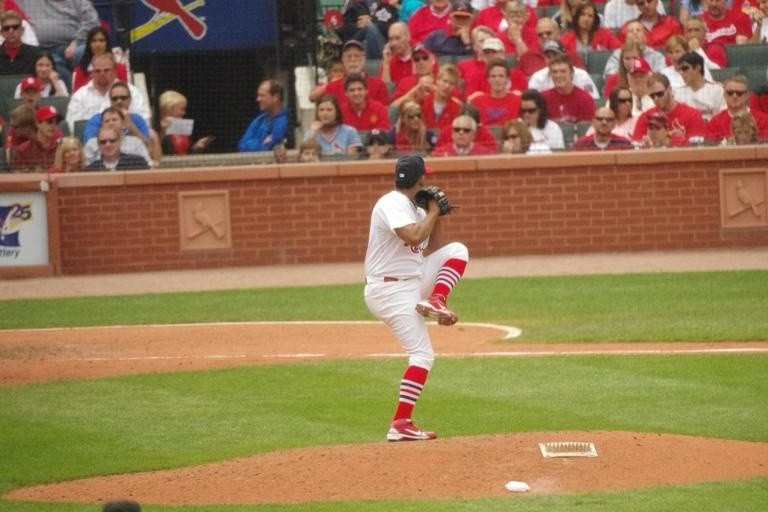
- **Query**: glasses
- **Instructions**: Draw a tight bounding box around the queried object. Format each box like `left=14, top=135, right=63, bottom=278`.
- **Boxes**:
left=454, top=128, right=474, bottom=134
left=407, top=112, right=422, bottom=119
left=3, top=24, right=22, bottom=31
left=520, top=108, right=538, bottom=113
left=617, top=99, right=632, bottom=102
left=96, top=68, right=113, bottom=74
left=649, top=89, right=664, bottom=98
left=414, top=55, right=431, bottom=61
left=727, top=90, right=747, bottom=96
left=111, top=95, right=130, bottom=100
left=679, top=66, right=690, bottom=72
left=638, top=0, right=654, bottom=5
left=505, top=135, right=521, bottom=139
left=596, top=117, right=615, bottom=122
left=537, top=31, right=554, bottom=38
left=99, top=138, right=120, bottom=144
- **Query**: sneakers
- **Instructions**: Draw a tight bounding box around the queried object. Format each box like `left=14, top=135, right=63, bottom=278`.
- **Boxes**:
left=418, top=297, right=458, bottom=324
left=386, top=418, right=438, bottom=441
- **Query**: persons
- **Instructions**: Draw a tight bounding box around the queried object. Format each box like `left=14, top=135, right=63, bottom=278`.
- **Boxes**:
left=529, top=40, right=602, bottom=107
left=338, top=75, right=392, bottom=133
left=66, top=51, right=150, bottom=134
left=698, top=0, right=751, bottom=45
left=553, top=0, right=604, bottom=30
left=420, top=0, right=474, bottom=56
left=13, top=105, right=65, bottom=172
left=519, top=89, right=566, bottom=150
left=560, top=3, right=621, bottom=70
left=405, top=0, right=455, bottom=41
left=460, top=38, right=528, bottom=101
left=704, top=75, right=768, bottom=144
left=659, top=36, right=690, bottom=94
left=357, top=129, right=391, bottom=160
left=384, top=101, right=437, bottom=156
left=675, top=54, right=730, bottom=121
left=469, top=0, right=510, bottom=34
left=436, top=103, right=497, bottom=150
left=495, top=3, right=538, bottom=58
left=538, top=56, right=598, bottom=121
left=587, top=86, right=640, bottom=139
left=1, top=10, right=55, bottom=76
left=500, top=119, right=551, bottom=154
left=388, top=44, right=438, bottom=113
left=518, top=17, right=587, bottom=72
left=603, top=0, right=668, bottom=30
left=49, top=136, right=86, bottom=172
left=237, top=79, right=295, bottom=152
left=603, top=21, right=668, bottom=79
left=13, top=50, right=70, bottom=98
left=13, top=0, right=101, bottom=93
left=83, top=126, right=149, bottom=171
left=750, top=1, right=768, bottom=44
left=574, top=108, right=635, bottom=150
left=365, top=153, right=468, bottom=441
left=420, top=64, right=464, bottom=134
left=605, top=44, right=646, bottom=99
left=468, top=65, right=522, bottom=127
left=430, top=116, right=494, bottom=157
left=310, top=57, right=345, bottom=101
left=683, top=16, right=728, bottom=70
left=617, top=1, right=684, bottom=47
left=274, top=140, right=321, bottom=164
left=83, top=107, right=155, bottom=167
left=457, top=24, right=495, bottom=77
left=325, top=38, right=388, bottom=106
left=641, top=113, right=691, bottom=148
left=605, top=57, right=660, bottom=116
left=5, top=78, right=43, bottom=148
left=334, top=0, right=399, bottom=59
left=378, top=22, right=424, bottom=84
left=83, top=82, right=152, bottom=151
left=156, top=91, right=216, bottom=156
left=303, top=95, right=365, bottom=159
left=397, top=0, right=427, bottom=23
left=721, top=110, right=764, bottom=145
left=632, top=74, right=704, bottom=144
left=73, top=25, right=128, bottom=92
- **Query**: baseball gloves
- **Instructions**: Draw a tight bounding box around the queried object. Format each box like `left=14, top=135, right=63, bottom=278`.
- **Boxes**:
left=413, top=185, right=453, bottom=217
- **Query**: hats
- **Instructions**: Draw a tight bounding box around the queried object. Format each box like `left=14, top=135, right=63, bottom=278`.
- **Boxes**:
left=449, top=2, right=474, bottom=18
left=411, top=47, right=432, bottom=56
left=343, top=39, right=364, bottom=51
left=482, top=37, right=506, bottom=53
left=646, top=112, right=670, bottom=127
left=21, top=76, right=44, bottom=93
left=628, top=54, right=652, bottom=76
left=36, top=104, right=65, bottom=121
left=394, top=154, right=433, bottom=180
left=366, top=129, right=389, bottom=145
left=542, top=38, right=565, bottom=55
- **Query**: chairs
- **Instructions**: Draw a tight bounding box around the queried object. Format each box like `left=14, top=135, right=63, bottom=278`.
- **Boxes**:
left=459, top=78, right=465, bottom=88
left=610, top=25, right=621, bottom=34
left=2, top=147, right=11, bottom=167
left=595, top=3, right=606, bottom=14
left=590, top=72, right=602, bottom=93
left=74, top=120, right=88, bottom=139
left=653, top=47, right=667, bottom=54
left=429, top=127, right=440, bottom=142
left=1, top=98, right=34, bottom=122
left=725, top=44, right=768, bottom=65
left=709, top=67, right=741, bottom=84
left=438, top=54, right=451, bottom=66
left=57, top=123, right=70, bottom=136
left=744, top=67, right=768, bottom=93
left=595, top=99, right=606, bottom=109
left=358, top=131, right=370, bottom=141
left=588, top=50, right=617, bottom=72
left=388, top=106, right=399, bottom=122
left=0, top=74, right=34, bottom=97
left=534, top=6, right=545, bottom=18
left=577, top=121, right=589, bottom=137
left=547, top=5, right=558, bottom=18
left=558, top=118, right=573, bottom=149
left=384, top=79, right=398, bottom=94
left=665, top=1, right=683, bottom=17
left=456, top=54, right=472, bottom=61
left=503, top=54, right=517, bottom=67
left=36, top=97, right=68, bottom=121
left=363, top=59, right=380, bottom=76
left=1, top=123, right=35, bottom=145
left=491, top=126, right=503, bottom=141
